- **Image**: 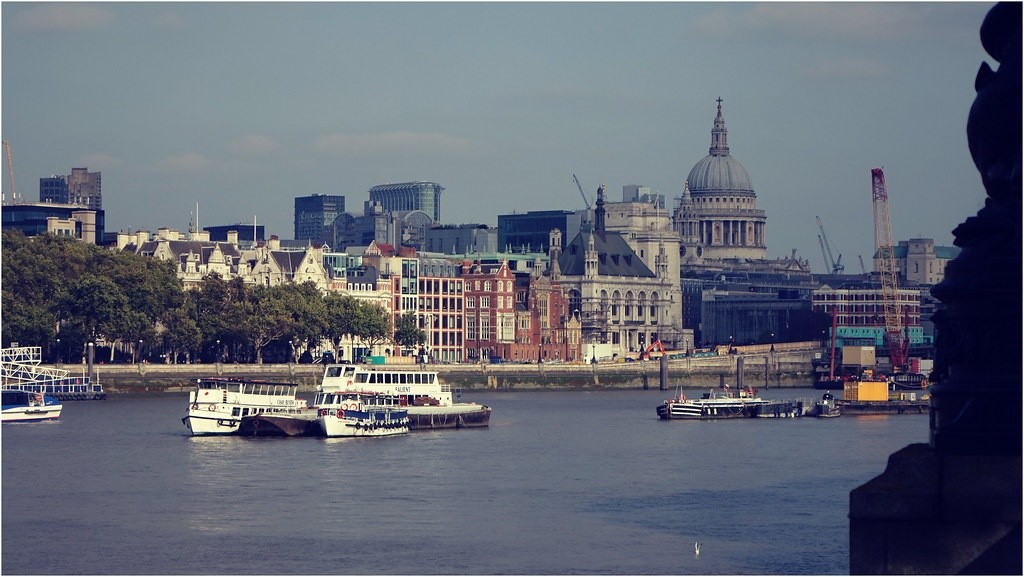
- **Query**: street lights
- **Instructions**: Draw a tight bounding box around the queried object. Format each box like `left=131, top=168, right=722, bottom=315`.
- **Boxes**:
left=139, top=340, right=143, bottom=363
left=217, top=339, right=220, bottom=364
left=686, top=339, right=690, bottom=357
left=57, top=338, right=61, bottom=363
left=537, top=343, right=542, bottom=364
left=421, top=343, right=425, bottom=364
left=357, top=342, right=360, bottom=363
left=770, top=334, right=774, bottom=352
left=640, top=341, right=644, bottom=360
left=591, top=344, right=598, bottom=364
left=729, top=335, right=732, bottom=354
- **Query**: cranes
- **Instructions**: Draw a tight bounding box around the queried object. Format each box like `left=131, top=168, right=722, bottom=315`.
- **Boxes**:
left=572, top=173, right=595, bottom=224
left=815, top=216, right=846, bottom=275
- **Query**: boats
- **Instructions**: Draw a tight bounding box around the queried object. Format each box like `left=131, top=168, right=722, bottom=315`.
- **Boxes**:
left=239, top=362, right=491, bottom=437
left=317, top=401, right=410, bottom=438
left=806, top=402, right=841, bottom=419
left=2, top=390, right=63, bottom=421
left=657, top=384, right=772, bottom=419
left=180, top=377, right=309, bottom=435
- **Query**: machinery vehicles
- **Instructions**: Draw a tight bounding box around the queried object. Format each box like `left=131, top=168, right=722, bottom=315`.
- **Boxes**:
left=639, top=340, right=666, bottom=361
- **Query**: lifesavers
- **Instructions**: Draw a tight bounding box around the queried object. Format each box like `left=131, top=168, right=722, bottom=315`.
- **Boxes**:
left=335, top=409, right=346, bottom=419
left=320, top=409, right=328, bottom=415
left=339, top=403, right=359, bottom=412
left=354, top=421, right=409, bottom=432
